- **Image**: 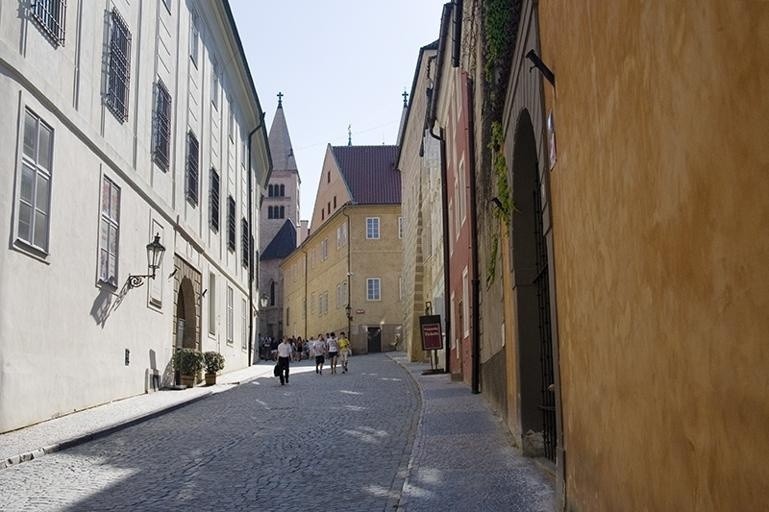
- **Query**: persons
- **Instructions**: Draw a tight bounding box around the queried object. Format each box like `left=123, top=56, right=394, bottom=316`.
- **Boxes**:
left=309, top=334, right=327, bottom=375
left=337, top=332, right=349, bottom=374
left=259, top=333, right=347, bottom=362
left=274, top=335, right=293, bottom=385
left=326, top=332, right=338, bottom=374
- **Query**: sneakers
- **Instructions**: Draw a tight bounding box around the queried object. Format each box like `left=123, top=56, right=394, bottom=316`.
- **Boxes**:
left=316, top=368, right=348, bottom=376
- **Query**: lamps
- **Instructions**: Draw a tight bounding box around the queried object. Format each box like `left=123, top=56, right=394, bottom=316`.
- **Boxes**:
left=126, top=232, right=166, bottom=287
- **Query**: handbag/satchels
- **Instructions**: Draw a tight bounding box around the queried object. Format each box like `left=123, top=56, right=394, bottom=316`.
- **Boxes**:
left=274, top=365, right=280, bottom=377
left=349, top=349, right=352, bottom=355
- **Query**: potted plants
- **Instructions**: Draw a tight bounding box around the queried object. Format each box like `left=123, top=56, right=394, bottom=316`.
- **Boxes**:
left=173, top=347, right=225, bottom=388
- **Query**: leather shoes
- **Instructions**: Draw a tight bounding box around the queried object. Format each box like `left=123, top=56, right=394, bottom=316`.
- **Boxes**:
left=280, top=379, right=289, bottom=385
